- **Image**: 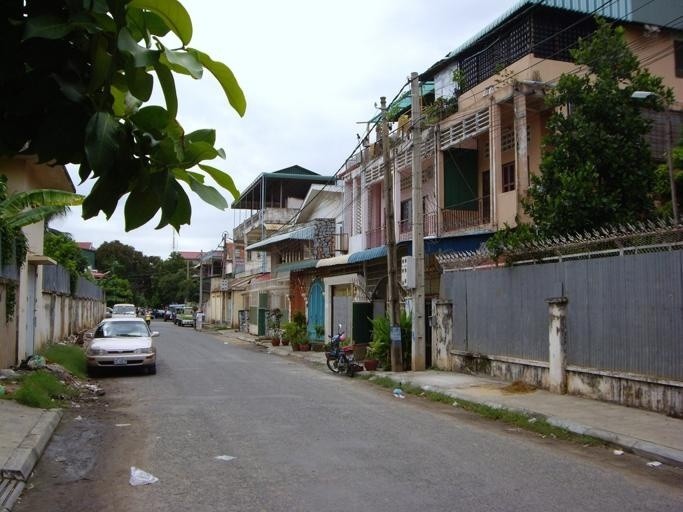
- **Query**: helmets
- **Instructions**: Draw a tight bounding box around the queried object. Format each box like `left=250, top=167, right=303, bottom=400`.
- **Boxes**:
left=338, top=334, right=345, bottom=342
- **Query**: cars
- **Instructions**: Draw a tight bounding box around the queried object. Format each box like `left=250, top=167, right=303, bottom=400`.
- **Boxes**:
left=83, top=317, right=160, bottom=379
left=106, top=303, right=197, bottom=328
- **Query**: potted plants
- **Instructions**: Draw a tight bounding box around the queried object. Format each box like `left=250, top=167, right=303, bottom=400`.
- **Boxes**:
left=362, top=339, right=384, bottom=371
left=269, top=310, right=326, bottom=351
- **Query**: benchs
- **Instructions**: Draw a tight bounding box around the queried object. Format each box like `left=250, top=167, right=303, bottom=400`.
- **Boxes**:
left=342, top=343, right=366, bottom=361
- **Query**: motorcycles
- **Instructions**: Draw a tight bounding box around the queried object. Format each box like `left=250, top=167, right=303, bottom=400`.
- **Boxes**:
left=325, top=323, right=356, bottom=378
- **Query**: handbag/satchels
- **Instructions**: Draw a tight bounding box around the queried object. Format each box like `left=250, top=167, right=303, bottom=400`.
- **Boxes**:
left=337, top=362, right=348, bottom=375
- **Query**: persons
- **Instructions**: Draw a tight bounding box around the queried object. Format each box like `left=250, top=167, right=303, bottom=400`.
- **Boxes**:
left=144, top=311, right=151, bottom=326
left=190, top=302, right=205, bottom=332
